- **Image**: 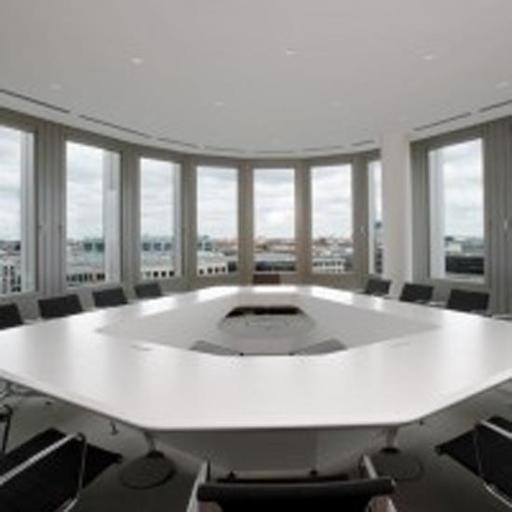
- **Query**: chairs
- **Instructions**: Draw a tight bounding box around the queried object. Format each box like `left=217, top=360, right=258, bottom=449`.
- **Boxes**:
left=364, top=271, right=494, bottom=317
left=433, top=411, right=512, bottom=511
left=1, top=398, right=120, bottom=512
left=182, top=453, right=401, bottom=512
left=250, top=274, right=282, bottom=284
left=0, top=279, right=162, bottom=328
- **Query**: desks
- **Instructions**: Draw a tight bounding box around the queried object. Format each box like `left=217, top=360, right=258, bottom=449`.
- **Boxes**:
left=0, top=284, right=510, bottom=486
